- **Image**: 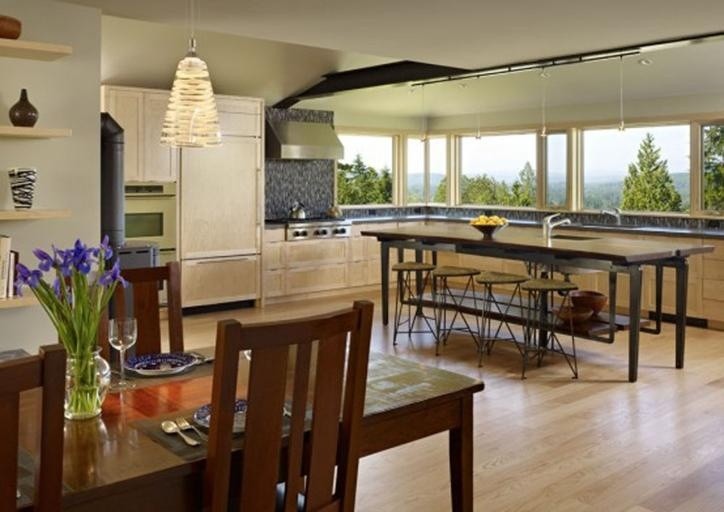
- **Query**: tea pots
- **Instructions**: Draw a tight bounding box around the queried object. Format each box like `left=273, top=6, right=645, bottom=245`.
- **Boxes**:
left=288, top=202, right=306, bottom=220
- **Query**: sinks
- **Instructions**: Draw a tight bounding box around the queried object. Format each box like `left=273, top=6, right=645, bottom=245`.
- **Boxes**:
left=583, top=224, right=646, bottom=228
left=553, top=235, right=599, bottom=241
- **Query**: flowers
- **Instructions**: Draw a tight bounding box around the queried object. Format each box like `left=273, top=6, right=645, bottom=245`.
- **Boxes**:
left=14, top=234, right=129, bottom=413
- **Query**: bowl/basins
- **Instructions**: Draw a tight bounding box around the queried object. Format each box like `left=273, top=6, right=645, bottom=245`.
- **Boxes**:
left=552, top=307, right=594, bottom=324
left=569, top=290, right=608, bottom=316
left=470, top=224, right=509, bottom=235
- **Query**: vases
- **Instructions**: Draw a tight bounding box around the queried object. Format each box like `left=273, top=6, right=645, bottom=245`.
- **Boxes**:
left=64, top=346, right=111, bottom=420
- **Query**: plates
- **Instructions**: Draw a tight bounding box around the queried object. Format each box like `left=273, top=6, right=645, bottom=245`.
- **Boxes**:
left=123, top=353, right=198, bottom=377
left=193, top=398, right=289, bottom=434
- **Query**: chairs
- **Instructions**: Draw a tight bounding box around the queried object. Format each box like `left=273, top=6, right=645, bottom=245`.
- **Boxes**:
left=100, top=262, right=183, bottom=363
left=0, top=345, right=66, bottom=512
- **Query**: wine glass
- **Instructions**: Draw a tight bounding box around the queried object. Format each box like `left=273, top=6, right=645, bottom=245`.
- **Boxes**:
left=108, top=317, right=139, bottom=391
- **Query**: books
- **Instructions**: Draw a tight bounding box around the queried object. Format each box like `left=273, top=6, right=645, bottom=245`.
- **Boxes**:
left=0, top=235, right=19, bottom=299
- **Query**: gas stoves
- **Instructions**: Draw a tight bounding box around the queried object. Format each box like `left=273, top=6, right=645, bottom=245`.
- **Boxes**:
left=265, top=215, right=353, bottom=241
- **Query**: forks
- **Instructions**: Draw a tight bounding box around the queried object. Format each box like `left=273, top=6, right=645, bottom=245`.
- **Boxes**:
left=174, top=417, right=209, bottom=443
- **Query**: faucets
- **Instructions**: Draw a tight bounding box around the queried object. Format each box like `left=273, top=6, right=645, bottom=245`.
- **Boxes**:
left=600, top=207, right=621, bottom=225
left=544, top=212, right=570, bottom=243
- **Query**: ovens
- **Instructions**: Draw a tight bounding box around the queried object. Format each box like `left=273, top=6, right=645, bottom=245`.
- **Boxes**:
left=124, top=182, right=179, bottom=307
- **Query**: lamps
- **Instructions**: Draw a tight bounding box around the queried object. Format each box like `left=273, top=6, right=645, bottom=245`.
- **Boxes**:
left=619, top=57, right=625, bottom=131
left=541, top=67, right=547, bottom=136
left=160, top=0, right=223, bottom=148
left=476, top=76, right=481, bottom=139
left=420, top=85, right=426, bottom=142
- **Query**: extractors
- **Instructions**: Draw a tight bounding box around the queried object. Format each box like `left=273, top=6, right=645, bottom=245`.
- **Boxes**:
left=265, top=119, right=344, bottom=161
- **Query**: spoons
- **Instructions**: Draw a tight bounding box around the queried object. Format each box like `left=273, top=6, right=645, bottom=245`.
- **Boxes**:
left=161, top=420, right=200, bottom=448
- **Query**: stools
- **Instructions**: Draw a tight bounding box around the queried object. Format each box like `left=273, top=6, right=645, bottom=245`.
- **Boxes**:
left=201, top=301, right=374, bottom=512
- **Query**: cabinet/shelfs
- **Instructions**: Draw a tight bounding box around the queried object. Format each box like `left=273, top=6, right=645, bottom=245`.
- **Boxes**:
left=176, top=94, right=265, bottom=308
left=101, top=86, right=177, bottom=183
left=0, top=39, right=73, bottom=309
